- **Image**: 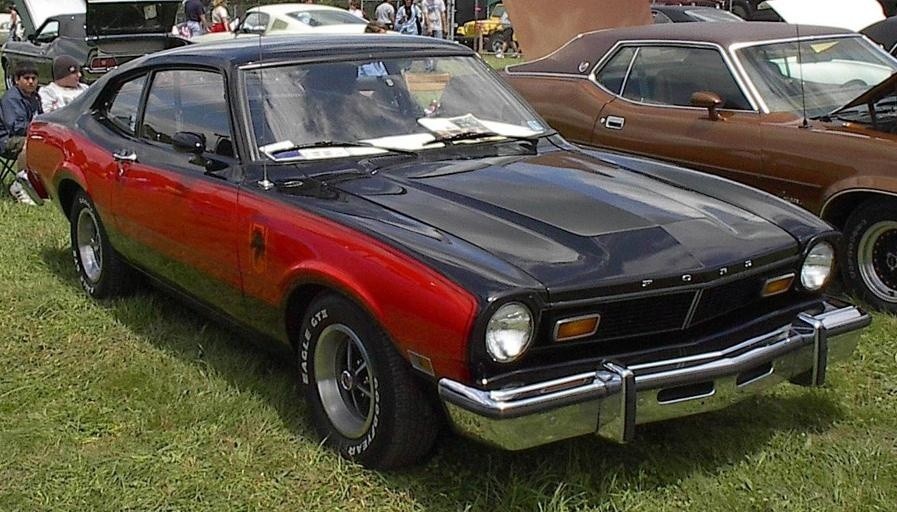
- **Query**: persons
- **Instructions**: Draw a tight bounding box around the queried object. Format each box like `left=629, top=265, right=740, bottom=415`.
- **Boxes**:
left=171, top=0, right=233, bottom=41
left=496, top=9, right=519, bottom=59
left=0, top=7, right=93, bottom=206
left=348, top=0, right=448, bottom=73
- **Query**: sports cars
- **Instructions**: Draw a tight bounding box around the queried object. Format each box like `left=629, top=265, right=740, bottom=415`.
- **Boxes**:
left=20, top=33, right=874, bottom=472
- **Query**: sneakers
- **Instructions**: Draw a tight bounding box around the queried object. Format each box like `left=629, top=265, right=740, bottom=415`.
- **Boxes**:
left=9, top=171, right=39, bottom=205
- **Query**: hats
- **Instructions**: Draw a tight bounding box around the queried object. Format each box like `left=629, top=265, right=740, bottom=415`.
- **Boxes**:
left=53, top=54, right=84, bottom=79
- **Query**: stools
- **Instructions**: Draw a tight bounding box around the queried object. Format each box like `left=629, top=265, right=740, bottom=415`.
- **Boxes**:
left=0, top=147, right=22, bottom=192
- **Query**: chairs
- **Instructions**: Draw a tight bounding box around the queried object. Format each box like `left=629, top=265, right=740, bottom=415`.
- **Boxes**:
left=304, top=62, right=381, bottom=146
left=653, top=69, right=721, bottom=109
